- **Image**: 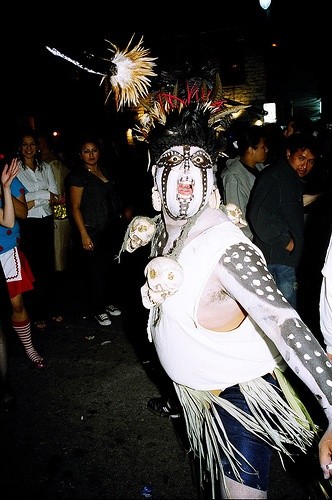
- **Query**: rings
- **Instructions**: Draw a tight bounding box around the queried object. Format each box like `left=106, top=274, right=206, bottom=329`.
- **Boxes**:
left=89, top=244, right=92, bottom=248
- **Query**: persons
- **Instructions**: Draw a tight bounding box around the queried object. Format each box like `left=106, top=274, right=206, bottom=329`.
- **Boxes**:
left=115, top=79, right=332, bottom=500
left=220, top=114, right=332, bottom=363
left=0, top=131, right=121, bottom=416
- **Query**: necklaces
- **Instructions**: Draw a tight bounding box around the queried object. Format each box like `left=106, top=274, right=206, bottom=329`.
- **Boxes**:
left=139, top=203, right=209, bottom=342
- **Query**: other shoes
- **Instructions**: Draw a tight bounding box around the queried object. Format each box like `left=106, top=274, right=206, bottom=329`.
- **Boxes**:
left=34, top=321, right=46, bottom=327
left=26, top=351, right=46, bottom=368
left=51, top=315, right=62, bottom=322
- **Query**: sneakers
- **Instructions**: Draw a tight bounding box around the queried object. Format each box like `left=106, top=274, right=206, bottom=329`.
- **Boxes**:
left=148, top=394, right=184, bottom=419
left=94, top=312, right=112, bottom=325
left=105, top=305, right=122, bottom=316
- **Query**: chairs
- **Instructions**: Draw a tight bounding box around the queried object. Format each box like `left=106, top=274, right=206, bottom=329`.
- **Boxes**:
left=49, top=159, right=73, bottom=271
